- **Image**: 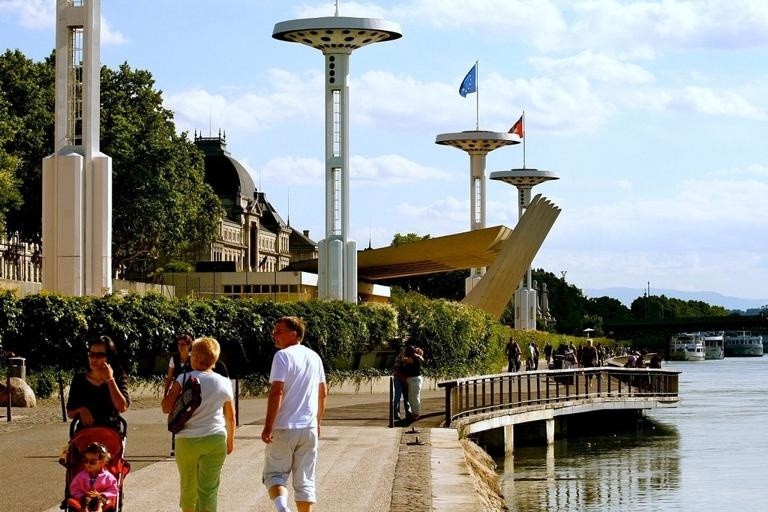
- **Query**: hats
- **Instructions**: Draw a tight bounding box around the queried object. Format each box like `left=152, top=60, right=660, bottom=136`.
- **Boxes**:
left=405, top=345, right=415, bottom=357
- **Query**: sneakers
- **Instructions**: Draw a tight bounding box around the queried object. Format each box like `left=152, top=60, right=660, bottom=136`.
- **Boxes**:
left=394, top=413, right=419, bottom=420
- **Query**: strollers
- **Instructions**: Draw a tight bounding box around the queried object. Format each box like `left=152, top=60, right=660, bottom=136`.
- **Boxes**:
left=59, top=412, right=131, bottom=512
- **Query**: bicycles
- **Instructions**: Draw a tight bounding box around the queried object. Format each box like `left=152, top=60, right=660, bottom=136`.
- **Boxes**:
left=508, top=352, right=521, bottom=383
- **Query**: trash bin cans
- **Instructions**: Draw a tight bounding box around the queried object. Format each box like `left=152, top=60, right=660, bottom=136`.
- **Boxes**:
left=7, top=357, right=26, bottom=380
left=554, top=354, right=565, bottom=369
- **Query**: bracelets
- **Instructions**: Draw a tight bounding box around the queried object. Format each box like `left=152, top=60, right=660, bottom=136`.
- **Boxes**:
left=105, top=377, right=115, bottom=384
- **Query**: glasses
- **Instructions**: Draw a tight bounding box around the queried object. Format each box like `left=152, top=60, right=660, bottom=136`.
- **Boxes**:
left=84, top=457, right=100, bottom=465
left=272, top=327, right=289, bottom=336
left=88, top=351, right=107, bottom=359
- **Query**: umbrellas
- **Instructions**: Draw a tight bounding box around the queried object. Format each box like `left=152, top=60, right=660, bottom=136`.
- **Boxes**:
left=583, top=327, right=595, bottom=337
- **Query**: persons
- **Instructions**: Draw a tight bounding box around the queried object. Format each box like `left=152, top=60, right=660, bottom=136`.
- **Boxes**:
left=506, top=336, right=664, bottom=396
left=261, top=315, right=328, bottom=512
left=66, top=335, right=130, bottom=436
left=393, top=348, right=424, bottom=420
left=401, top=345, right=424, bottom=419
left=161, top=338, right=237, bottom=511
left=69, top=441, right=120, bottom=512
left=163, top=334, right=194, bottom=458
left=183, top=358, right=231, bottom=381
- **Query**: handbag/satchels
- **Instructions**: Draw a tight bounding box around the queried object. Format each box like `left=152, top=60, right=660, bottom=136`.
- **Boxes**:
left=168, top=370, right=202, bottom=435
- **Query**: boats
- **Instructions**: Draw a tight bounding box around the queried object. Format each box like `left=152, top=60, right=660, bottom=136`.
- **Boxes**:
left=669, top=330, right=764, bottom=361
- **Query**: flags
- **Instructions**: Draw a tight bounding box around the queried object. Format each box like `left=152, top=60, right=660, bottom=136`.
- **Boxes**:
left=458, top=64, right=477, bottom=98
left=509, top=116, right=524, bottom=139
